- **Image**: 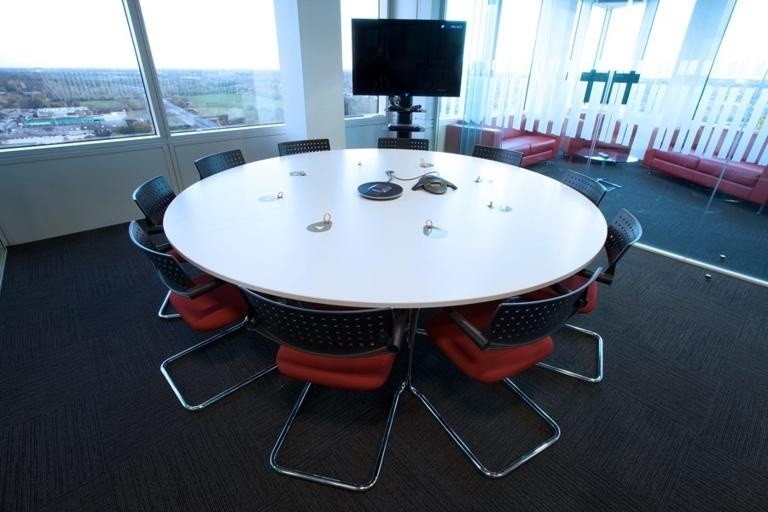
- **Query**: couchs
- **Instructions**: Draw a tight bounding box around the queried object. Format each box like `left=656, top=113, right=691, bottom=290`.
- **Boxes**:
left=642, top=121, right=767, bottom=214
left=444, top=107, right=639, bottom=170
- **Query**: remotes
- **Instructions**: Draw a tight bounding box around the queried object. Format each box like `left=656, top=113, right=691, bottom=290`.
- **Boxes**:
left=388, top=124, right=421, bottom=131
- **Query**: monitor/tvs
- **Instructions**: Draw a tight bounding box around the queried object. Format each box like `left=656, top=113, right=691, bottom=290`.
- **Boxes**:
left=351, top=19, right=467, bottom=97
